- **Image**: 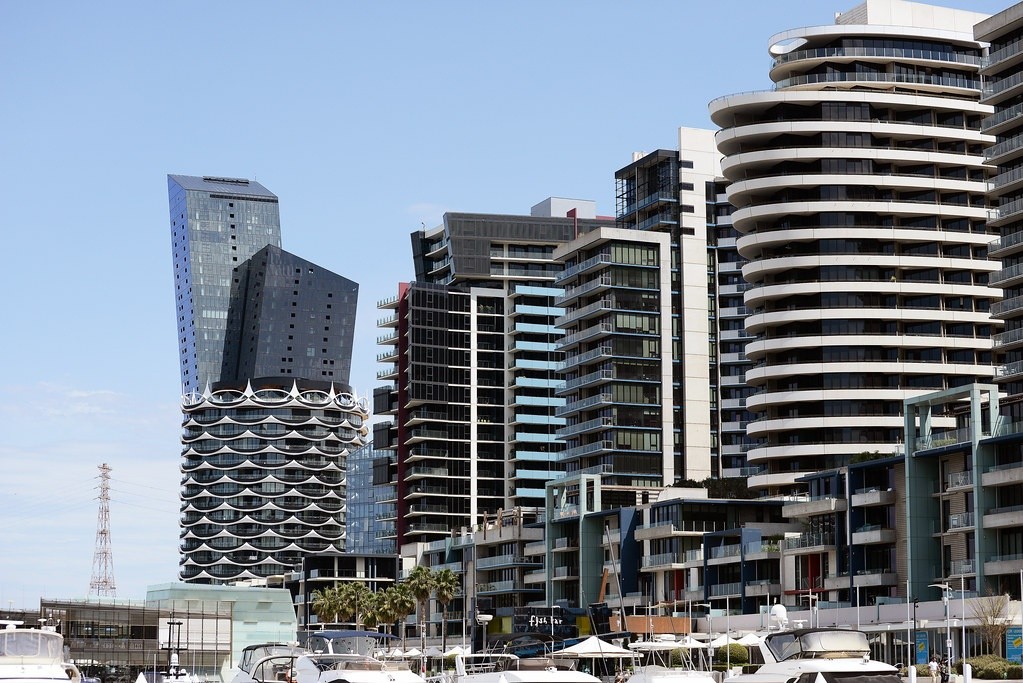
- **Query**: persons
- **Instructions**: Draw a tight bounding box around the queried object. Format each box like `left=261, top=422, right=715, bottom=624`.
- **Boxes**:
left=927, top=656, right=949, bottom=683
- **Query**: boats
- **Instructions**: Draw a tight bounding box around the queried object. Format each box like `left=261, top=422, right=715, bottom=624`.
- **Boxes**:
left=0, top=525, right=908, bottom=683
